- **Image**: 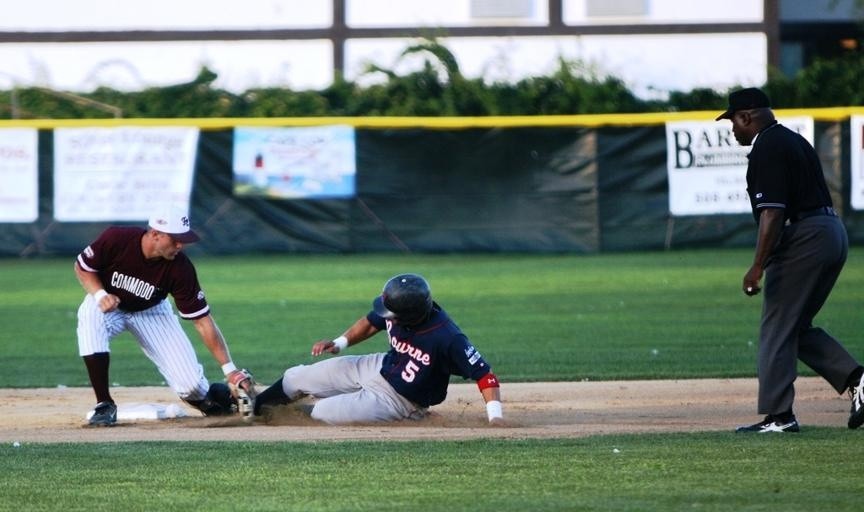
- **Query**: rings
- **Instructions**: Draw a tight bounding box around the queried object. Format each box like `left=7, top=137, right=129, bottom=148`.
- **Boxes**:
left=746, top=285, right=753, bottom=293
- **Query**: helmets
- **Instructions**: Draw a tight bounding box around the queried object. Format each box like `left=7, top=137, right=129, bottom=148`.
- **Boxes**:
left=373, top=275, right=433, bottom=325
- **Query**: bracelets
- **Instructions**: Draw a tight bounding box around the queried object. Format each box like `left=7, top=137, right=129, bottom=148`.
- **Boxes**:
left=486, top=399, right=504, bottom=423
left=332, top=336, right=349, bottom=350
left=220, top=360, right=237, bottom=376
left=94, top=289, right=109, bottom=301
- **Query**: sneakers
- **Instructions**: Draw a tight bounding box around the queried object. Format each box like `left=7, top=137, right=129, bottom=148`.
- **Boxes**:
left=847, top=370, right=864, bottom=430
left=89, top=401, right=118, bottom=426
left=736, top=416, right=799, bottom=433
left=203, top=383, right=237, bottom=416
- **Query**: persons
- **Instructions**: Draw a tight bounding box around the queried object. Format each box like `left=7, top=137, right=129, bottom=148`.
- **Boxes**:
left=208, top=272, right=523, bottom=430
left=73, top=208, right=257, bottom=429
left=714, top=88, right=864, bottom=439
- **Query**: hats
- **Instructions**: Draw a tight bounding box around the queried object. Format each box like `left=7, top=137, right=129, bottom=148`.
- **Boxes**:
left=716, top=88, right=769, bottom=121
left=149, top=206, right=201, bottom=243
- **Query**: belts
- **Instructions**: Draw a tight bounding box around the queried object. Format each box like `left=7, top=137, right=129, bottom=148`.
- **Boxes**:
left=798, top=205, right=838, bottom=218
